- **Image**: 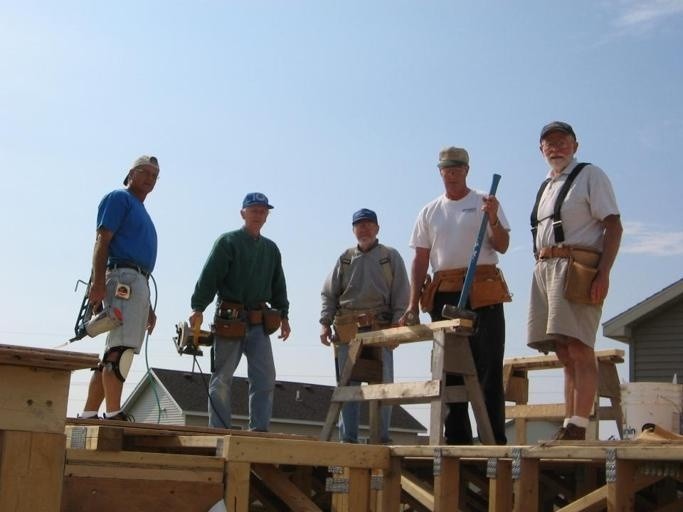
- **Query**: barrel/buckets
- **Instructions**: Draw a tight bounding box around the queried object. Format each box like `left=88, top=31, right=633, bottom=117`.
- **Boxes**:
left=618, top=382, right=683, bottom=438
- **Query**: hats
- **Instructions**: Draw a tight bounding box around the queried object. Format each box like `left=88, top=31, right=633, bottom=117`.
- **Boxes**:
left=243, top=193, right=273, bottom=209
left=123, top=155, right=159, bottom=186
left=437, top=147, right=469, bottom=168
left=352, top=209, right=377, bottom=224
left=540, top=121, right=575, bottom=142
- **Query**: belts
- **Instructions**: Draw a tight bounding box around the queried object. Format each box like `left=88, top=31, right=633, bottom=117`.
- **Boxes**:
left=109, top=262, right=149, bottom=279
left=539, top=247, right=570, bottom=259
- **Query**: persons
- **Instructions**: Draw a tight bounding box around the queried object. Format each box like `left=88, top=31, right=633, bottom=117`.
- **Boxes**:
left=316, top=207, right=411, bottom=447
left=188, top=192, right=292, bottom=432
left=524, top=121, right=623, bottom=439
left=75, top=153, right=160, bottom=421
left=398, top=146, right=514, bottom=445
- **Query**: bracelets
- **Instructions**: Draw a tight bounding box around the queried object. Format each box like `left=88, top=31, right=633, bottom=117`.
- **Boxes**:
left=490, top=217, right=500, bottom=229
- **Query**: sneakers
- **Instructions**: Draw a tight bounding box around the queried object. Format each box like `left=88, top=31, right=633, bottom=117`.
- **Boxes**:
left=553, top=423, right=585, bottom=440
left=76, top=411, right=126, bottom=420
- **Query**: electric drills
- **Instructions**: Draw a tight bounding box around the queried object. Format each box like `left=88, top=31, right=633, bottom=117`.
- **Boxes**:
left=173, top=317, right=213, bottom=379
left=56, top=301, right=123, bottom=348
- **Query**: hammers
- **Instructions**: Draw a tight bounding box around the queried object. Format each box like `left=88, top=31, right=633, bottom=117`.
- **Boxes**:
left=441, top=174, right=502, bottom=324
